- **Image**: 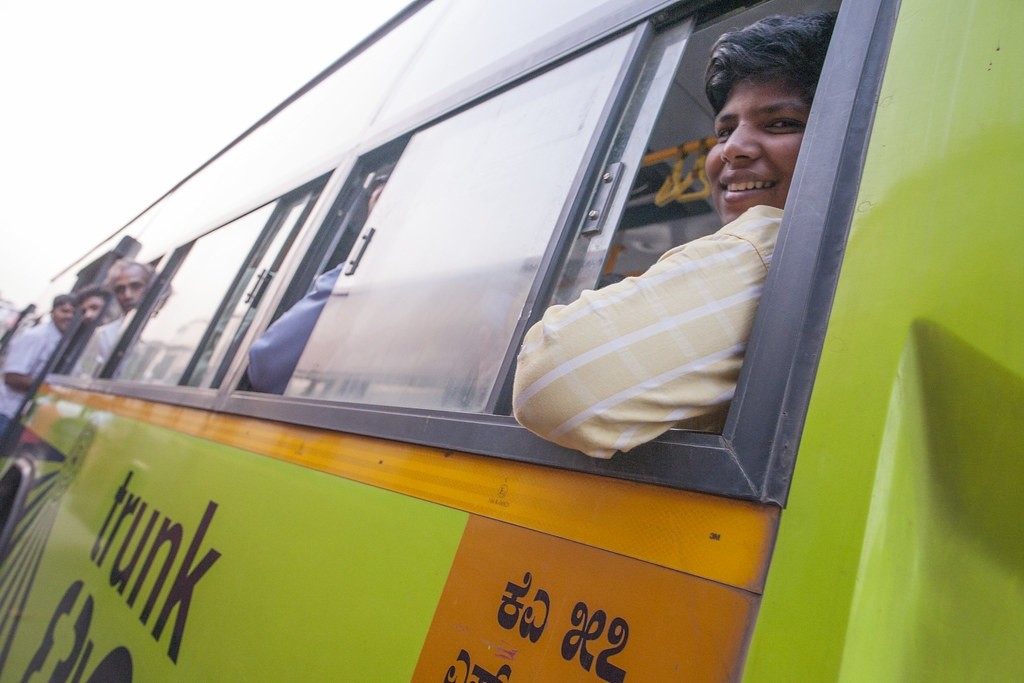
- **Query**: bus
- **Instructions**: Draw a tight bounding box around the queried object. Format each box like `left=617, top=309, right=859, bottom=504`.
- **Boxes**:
left=0, top=0, right=1024, bottom=683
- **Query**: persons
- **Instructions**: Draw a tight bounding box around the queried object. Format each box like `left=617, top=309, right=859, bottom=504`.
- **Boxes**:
left=246, top=163, right=398, bottom=395
left=0, top=259, right=154, bottom=455
left=512, top=13, right=839, bottom=460
